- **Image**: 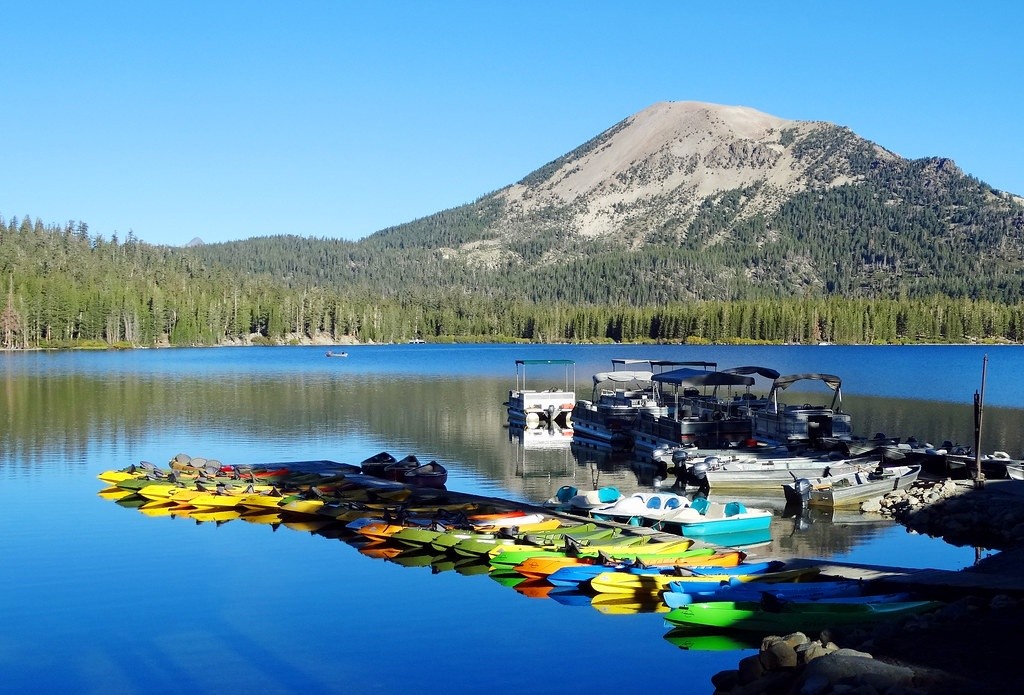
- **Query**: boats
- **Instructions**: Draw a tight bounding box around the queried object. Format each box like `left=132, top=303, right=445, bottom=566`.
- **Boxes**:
left=326, top=350, right=349, bottom=357
left=505, top=359, right=577, bottom=431
left=95, top=489, right=766, bottom=650
left=95, top=452, right=947, bottom=636
left=570, top=359, right=854, bottom=451
